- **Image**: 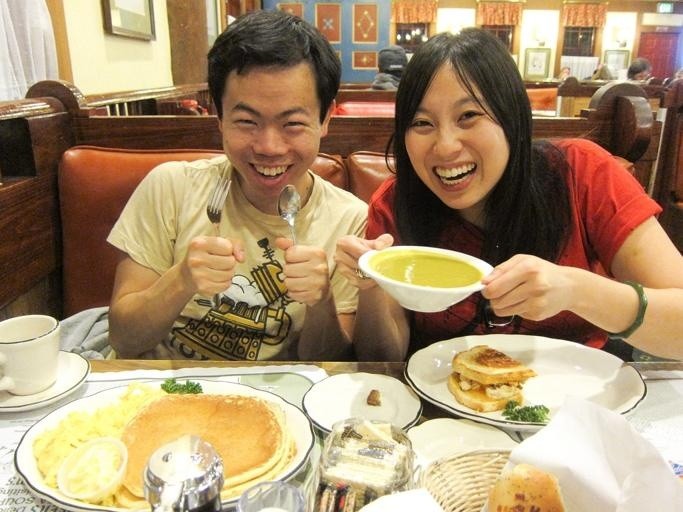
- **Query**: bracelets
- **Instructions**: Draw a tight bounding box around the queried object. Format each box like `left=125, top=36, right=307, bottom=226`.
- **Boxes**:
left=607, top=280, right=647, bottom=340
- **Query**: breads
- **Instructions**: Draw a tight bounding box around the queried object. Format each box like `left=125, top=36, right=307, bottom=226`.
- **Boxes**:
left=488, top=464, right=568, bottom=512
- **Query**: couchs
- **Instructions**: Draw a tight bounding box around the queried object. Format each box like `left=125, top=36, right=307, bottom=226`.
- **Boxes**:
left=331, top=101, right=396, bottom=116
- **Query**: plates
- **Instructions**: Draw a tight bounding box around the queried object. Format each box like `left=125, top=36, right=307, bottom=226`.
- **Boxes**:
left=13, top=378, right=313, bottom=512
left=0, top=351, right=90, bottom=414
left=405, top=335, right=647, bottom=434
left=399, top=416, right=524, bottom=494
left=300, top=373, right=424, bottom=442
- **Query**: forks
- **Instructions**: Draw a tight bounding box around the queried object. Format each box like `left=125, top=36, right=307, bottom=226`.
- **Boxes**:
left=207, top=176, right=232, bottom=309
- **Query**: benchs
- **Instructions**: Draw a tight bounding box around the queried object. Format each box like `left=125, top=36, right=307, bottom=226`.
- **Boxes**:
left=56, top=143, right=635, bottom=321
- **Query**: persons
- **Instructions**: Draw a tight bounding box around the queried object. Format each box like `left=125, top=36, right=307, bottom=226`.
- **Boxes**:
left=333, top=27, right=683, bottom=363
left=559, top=67, right=570, bottom=80
left=105, top=8, right=369, bottom=359
left=627, top=58, right=653, bottom=80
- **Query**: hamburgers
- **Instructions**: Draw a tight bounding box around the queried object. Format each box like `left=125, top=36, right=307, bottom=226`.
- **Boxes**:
left=448, top=345, right=537, bottom=413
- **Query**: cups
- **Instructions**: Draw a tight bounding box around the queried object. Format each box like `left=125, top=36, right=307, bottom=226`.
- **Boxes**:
left=0, top=314, right=60, bottom=398
left=236, top=481, right=308, bottom=512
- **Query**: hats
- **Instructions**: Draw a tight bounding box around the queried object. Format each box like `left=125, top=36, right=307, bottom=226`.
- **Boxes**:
left=378, top=45, right=408, bottom=72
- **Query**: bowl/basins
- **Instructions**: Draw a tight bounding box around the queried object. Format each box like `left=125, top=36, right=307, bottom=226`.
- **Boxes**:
left=56, top=434, right=129, bottom=504
left=355, top=245, right=496, bottom=314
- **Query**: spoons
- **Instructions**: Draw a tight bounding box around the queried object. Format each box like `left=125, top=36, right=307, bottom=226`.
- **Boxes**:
left=277, top=184, right=302, bottom=308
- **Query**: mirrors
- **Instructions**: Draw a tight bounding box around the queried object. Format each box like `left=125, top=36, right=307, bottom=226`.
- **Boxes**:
left=100, top=0, right=157, bottom=41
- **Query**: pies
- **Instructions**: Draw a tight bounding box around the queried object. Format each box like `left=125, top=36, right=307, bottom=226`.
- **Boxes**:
left=118, top=393, right=287, bottom=487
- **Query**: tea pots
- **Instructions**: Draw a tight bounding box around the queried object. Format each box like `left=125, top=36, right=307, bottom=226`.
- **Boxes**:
left=143, top=436, right=225, bottom=511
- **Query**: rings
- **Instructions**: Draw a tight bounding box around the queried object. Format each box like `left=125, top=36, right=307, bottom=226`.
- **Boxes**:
left=355, top=268, right=370, bottom=280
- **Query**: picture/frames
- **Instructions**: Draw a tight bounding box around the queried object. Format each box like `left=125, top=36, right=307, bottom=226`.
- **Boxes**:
left=315, top=2, right=342, bottom=44
left=604, top=50, right=630, bottom=69
left=523, top=48, right=552, bottom=80
left=276, top=3, right=304, bottom=18
left=352, top=51, right=379, bottom=70
left=352, top=3, right=379, bottom=44
